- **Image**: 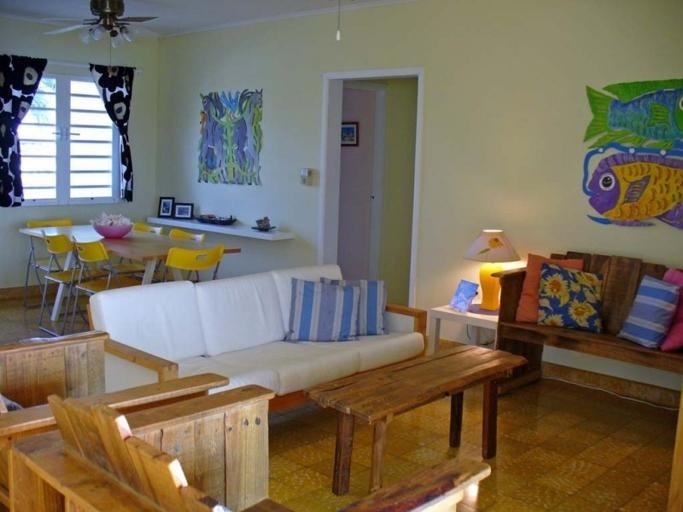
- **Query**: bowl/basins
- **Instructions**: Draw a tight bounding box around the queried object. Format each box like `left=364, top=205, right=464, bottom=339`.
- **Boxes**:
left=92, top=224, right=132, bottom=239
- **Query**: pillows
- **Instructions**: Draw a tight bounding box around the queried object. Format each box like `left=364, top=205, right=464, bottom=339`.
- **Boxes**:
left=616, top=274, right=681, bottom=349
left=320, top=277, right=386, bottom=335
left=659, top=267, right=683, bottom=352
left=537, top=262, right=608, bottom=333
left=516, top=254, right=584, bottom=323
left=283, top=278, right=360, bottom=342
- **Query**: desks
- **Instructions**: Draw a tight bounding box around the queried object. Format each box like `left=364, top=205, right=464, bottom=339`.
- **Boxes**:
left=428, top=300, right=499, bottom=354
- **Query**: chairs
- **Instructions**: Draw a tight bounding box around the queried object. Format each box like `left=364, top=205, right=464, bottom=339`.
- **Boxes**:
left=9, top=384, right=491, bottom=512
left=0, top=331, right=229, bottom=512
left=18, top=218, right=225, bottom=333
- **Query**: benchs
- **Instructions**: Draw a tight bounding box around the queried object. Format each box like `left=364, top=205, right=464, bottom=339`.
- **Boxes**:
left=305, top=345, right=529, bottom=497
left=491, top=268, right=683, bottom=396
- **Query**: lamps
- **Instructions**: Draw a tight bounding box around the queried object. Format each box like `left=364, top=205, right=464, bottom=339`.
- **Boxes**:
left=335, top=1, right=342, bottom=42
left=79, top=24, right=135, bottom=48
left=463, top=229, right=521, bottom=311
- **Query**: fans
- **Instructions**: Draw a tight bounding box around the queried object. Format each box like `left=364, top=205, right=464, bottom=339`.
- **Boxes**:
left=41, top=0, right=159, bottom=35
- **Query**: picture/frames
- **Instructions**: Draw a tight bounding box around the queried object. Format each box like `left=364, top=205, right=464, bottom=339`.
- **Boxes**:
left=341, top=122, right=359, bottom=146
left=174, top=203, right=193, bottom=219
left=158, top=197, right=175, bottom=218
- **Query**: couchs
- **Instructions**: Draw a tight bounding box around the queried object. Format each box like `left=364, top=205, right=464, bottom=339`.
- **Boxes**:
left=86, top=264, right=427, bottom=412
